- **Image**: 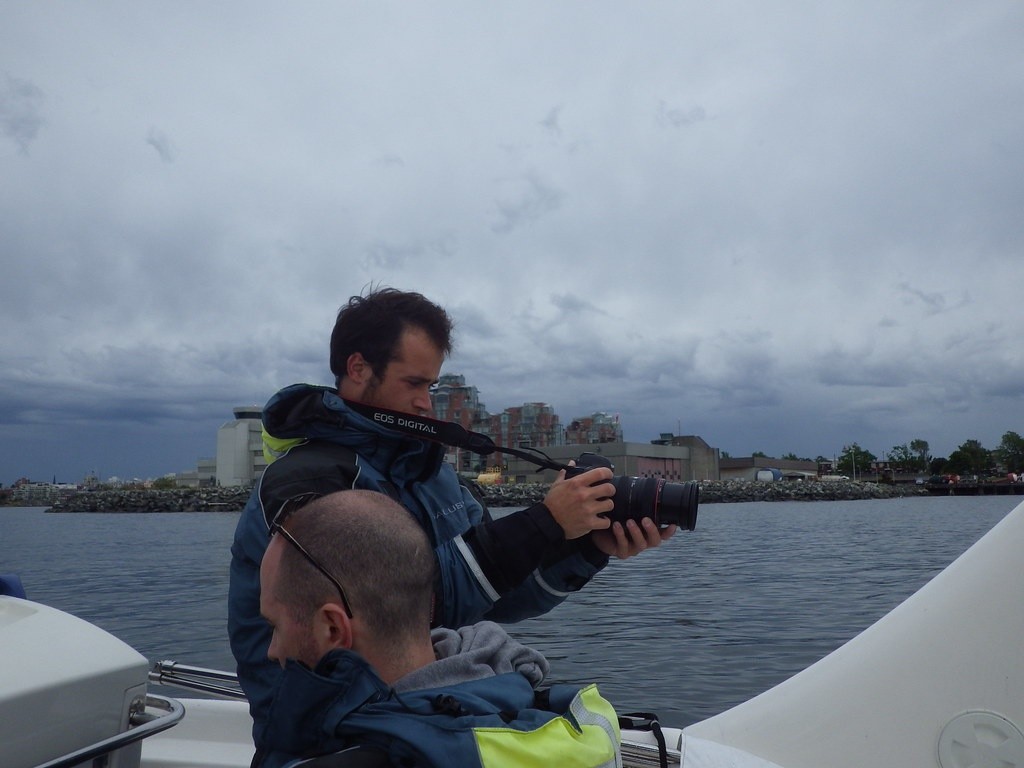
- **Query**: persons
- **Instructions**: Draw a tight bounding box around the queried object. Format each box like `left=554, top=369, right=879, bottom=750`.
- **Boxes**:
left=251, top=491, right=621, bottom=768
left=229, top=287, right=677, bottom=751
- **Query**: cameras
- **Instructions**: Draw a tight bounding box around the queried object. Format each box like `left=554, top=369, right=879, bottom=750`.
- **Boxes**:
left=567, top=452, right=699, bottom=534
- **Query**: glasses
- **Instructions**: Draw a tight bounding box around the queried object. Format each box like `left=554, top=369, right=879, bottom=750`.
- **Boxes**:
left=266, top=492, right=353, bottom=620
